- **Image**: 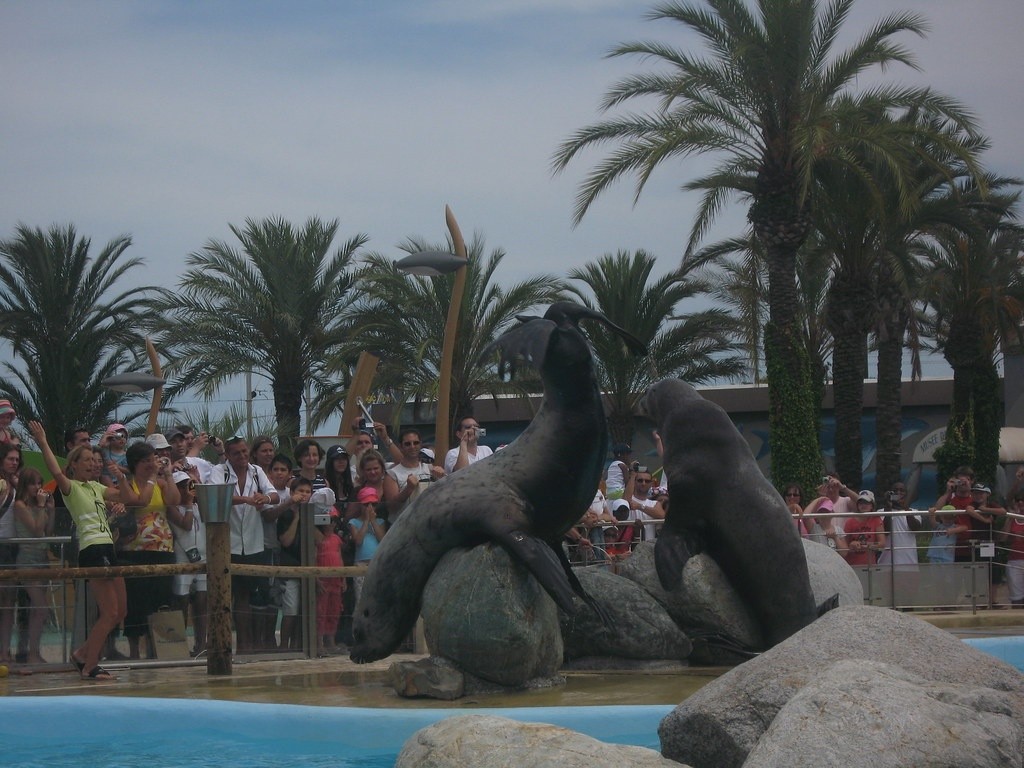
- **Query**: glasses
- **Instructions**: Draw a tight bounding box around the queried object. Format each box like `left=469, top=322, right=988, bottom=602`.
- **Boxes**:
left=1016, top=496, right=1024, bottom=501
left=418, top=474, right=436, bottom=483
left=402, top=440, right=421, bottom=447
left=225, top=436, right=244, bottom=449
left=784, top=493, right=800, bottom=498
left=636, top=478, right=654, bottom=484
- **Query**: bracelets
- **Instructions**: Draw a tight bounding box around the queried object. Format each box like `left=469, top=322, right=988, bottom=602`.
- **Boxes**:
left=147, top=480, right=155, bottom=485
left=839, top=484, right=846, bottom=490
left=266, top=494, right=272, bottom=504
left=216, top=448, right=225, bottom=456
left=576, top=537, right=582, bottom=542
left=185, top=509, right=194, bottom=512
left=118, top=474, right=126, bottom=480
left=38, top=505, right=46, bottom=509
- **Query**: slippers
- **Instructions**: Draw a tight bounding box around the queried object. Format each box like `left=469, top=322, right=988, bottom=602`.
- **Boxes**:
left=69, top=655, right=85, bottom=674
left=82, top=666, right=116, bottom=682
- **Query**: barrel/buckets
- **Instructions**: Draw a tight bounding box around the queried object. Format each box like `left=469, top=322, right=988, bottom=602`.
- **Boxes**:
left=194, top=482, right=237, bottom=523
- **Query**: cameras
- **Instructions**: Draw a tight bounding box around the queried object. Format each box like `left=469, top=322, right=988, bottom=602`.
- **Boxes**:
left=113, top=432, right=124, bottom=441
left=632, top=461, right=648, bottom=472
left=161, top=458, right=169, bottom=468
left=208, top=434, right=216, bottom=443
left=45, top=492, right=51, bottom=501
left=359, top=419, right=375, bottom=429
left=475, top=428, right=486, bottom=436
left=822, top=476, right=831, bottom=484
left=888, top=490, right=901, bottom=501
left=188, top=480, right=196, bottom=490
left=954, top=479, right=962, bottom=485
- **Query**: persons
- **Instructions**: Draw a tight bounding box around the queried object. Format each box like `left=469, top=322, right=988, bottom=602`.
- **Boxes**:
left=781, top=464, right=1024, bottom=611
left=0, top=399, right=101, bottom=668
left=26, top=421, right=131, bottom=682
left=559, top=430, right=681, bottom=561
left=98, top=416, right=495, bottom=670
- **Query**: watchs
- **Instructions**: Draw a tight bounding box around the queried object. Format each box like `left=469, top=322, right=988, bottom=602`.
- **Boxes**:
left=640, top=504, right=647, bottom=512
left=385, top=439, right=393, bottom=446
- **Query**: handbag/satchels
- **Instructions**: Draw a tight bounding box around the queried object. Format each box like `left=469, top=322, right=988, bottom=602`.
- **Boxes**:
left=108, top=506, right=137, bottom=536
left=186, top=546, right=202, bottom=564
left=998, top=516, right=1014, bottom=544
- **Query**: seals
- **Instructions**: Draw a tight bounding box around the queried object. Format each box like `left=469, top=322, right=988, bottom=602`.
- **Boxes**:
left=640, top=380, right=839, bottom=658
left=348, top=298, right=649, bottom=664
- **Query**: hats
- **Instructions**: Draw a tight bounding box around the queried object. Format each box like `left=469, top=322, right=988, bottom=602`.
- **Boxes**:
left=0, top=400, right=16, bottom=417
left=613, top=498, right=630, bottom=511
left=145, top=433, right=172, bottom=450
left=614, top=442, right=632, bottom=455
left=420, top=448, right=435, bottom=459
left=106, top=423, right=128, bottom=442
left=939, top=505, right=956, bottom=516
left=326, top=445, right=349, bottom=458
left=814, top=498, right=835, bottom=512
left=856, top=490, right=876, bottom=505
left=357, top=487, right=378, bottom=504
left=971, top=481, right=991, bottom=495
left=165, top=429, right=184, bottom=442
left=323, top=505, right=339, bottom=520
left=172, top=471, right=190, bottom=484
